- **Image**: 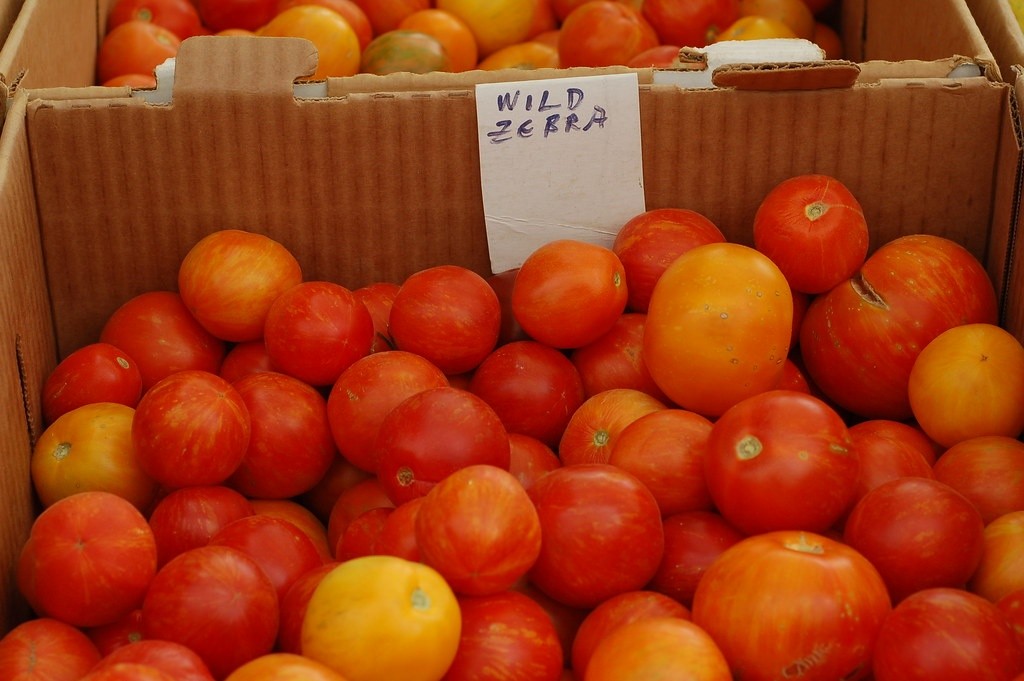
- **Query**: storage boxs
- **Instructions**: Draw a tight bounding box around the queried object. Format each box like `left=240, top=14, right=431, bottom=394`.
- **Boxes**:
left=0, top=0, right=1024, bottom=633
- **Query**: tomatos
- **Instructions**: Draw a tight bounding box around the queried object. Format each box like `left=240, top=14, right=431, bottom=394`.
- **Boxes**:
left=0, top=171, right=1023, bottom=681
left=95, top=0, right=847, bottom=91
